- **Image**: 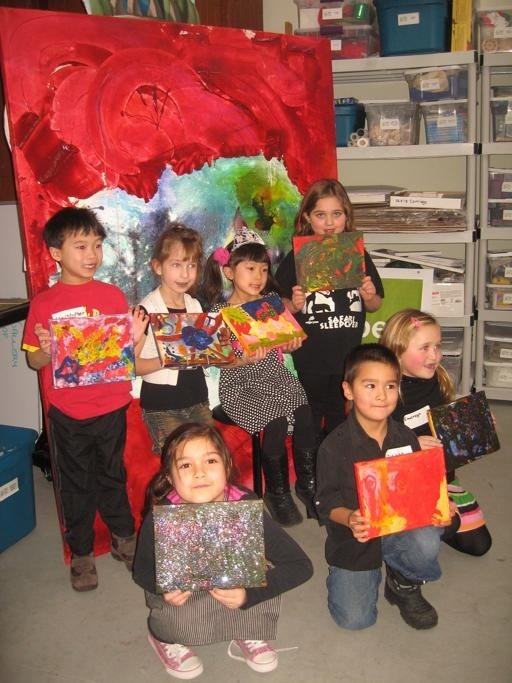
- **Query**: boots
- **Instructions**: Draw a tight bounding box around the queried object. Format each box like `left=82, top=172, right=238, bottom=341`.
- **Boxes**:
left=295, top=446, right=318, bottom=520
left=262, top=455, right=303, bottom=527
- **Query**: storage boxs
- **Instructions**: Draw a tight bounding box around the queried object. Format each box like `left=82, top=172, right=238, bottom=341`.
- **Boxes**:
left=420, top=104, right=468, bottom=145
left=0, top=424, right=40, bottom=558
left=490, top=201, right=511, bottom=226
left=372, top=0, right=451, bottom=56
left=361, top=103, right=418, bottom=148
left=484, top=335, right=512, bottom=365
left=489, top=167, right=512, bottom=199
left=490, top=97, right=512, bottom=143
left=483, top=363, right=510, bottom=386
left=489, top=72, right=512, bottom=88
left=487, top=251, right=512, bottom=284
left=399, top=66, right=468, bottom=103
left=339, top=24, right=379, bottom=58
left=490, top=287, right=512, bottom=311
left=333, top=97, right=366, bottom=146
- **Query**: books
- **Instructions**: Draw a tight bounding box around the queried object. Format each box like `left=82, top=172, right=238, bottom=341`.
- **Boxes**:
left=387, top=245, right=466, bottom=281
left=388, top=191, right=468, bottom=211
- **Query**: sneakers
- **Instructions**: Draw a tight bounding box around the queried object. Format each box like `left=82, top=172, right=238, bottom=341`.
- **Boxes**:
left=384, top=575, right=438, bottom=630
left=147, top=630, right=203, bottom=680
left=226, top=640, right=298, bottom=674
left=110, top=532, right=137, bottom=573
left=70, top=550, right=99, bottom=592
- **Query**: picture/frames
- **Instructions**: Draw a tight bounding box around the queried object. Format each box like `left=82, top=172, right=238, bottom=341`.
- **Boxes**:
left=354, top=446, right=450, bottom=541
left=292, top=231, right=367, bottom=295
left=148, top=311, right=241, bottom=366
left=154, top=500, right=268, bottom=597
left=47, top=312, right=136, bottom=387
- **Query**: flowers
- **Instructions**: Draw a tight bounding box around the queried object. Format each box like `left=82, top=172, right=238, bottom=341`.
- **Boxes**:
left=220, top=292, right=309, bottom=356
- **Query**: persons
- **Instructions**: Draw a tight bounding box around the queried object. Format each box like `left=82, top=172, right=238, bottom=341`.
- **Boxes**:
left=133, top=421, right=315, bottom=680
left=206, top=230, right=307, bottom=530
left=315, top=346, right=445, bottom=632
left=275, top=180, right=388, bottom=491
left=126, top=220, right=221, bottom=459
left=21, top=208, right=150, bottom=591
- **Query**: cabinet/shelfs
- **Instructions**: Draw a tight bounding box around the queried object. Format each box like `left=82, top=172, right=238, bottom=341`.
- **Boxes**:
left=481, top=51, right=512, bottom=402
left=333, top=52, right=474, bottom=394
